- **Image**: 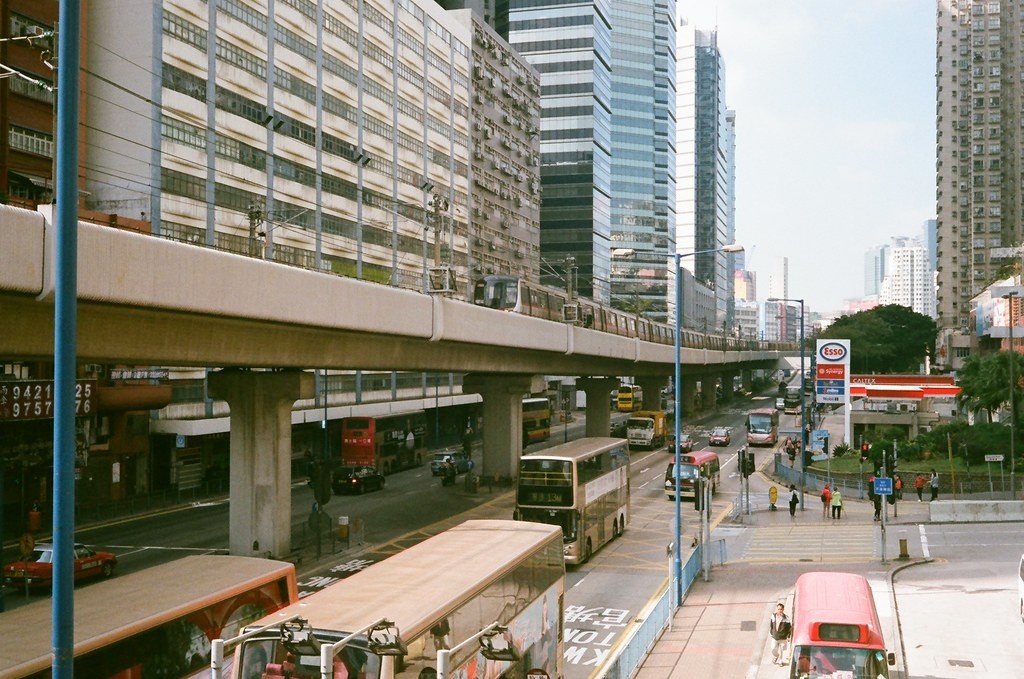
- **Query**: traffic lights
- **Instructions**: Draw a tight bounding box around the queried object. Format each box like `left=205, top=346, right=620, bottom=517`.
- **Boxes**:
left=861, top=443, right=869, bottom=460
left=805, top=451, right=814, bottom=466
left=872, top=457, right=898, bottom=478
left=822, top=437, right=828, bottom=454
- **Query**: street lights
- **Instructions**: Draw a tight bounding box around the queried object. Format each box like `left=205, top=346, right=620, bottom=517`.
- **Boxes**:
left=613, top=243, right=745, bottom=600
left=767, top=297, right=806, bottom=471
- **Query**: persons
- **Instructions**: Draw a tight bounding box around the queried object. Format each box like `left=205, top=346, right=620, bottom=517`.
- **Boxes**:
left=788, top=484, right=799, bottom=517
left=430, top=617, right=451, bottom=651
left=463, top=428, right=473, bottom=459
left=444, top=458, right=455, bottom=476
left=784, top=435, right=801, bottom=469
left=769, top=603, right=792, bottom=667
left=874, top=502, right=881, bottom=521
left=895, top=475, right=902, bottom=501
left=822, top=484, right=842, bottom=520
left=915, top=469, right=939, bottom=502
left=868, top=441, right=873, bottom=450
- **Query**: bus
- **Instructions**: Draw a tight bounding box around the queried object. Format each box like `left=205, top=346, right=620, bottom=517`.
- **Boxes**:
left=338, top=407, right=427, bottom=473
left=664, top=451, right=720, bottom=501
left=783, top=377, right=813, bottom=414
left=520, top=382, right=644, bottom=446
left=744, top=409, right=780, bottom=445
left=0, top=518, right=564, bottom=679
left=788, top=571, right=896, bottom=679
left=514, top=435, right=634, bottom=567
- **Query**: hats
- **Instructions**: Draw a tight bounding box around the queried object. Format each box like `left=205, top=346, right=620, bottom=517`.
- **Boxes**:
left=790, top=484, right=795, bottom=488
left=825, top=484, right=829, bottom=488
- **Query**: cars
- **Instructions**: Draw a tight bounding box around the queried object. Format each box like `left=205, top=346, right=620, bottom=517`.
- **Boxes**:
left=1, top=542, right=117, bottom=595
left=774, top=398, right=784, bottom=410
left=331, top=448, right=473, bottom=495
left=667, top=426, right=731, bottom=453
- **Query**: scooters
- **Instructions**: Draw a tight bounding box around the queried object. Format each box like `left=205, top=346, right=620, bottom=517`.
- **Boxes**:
left=438, top=461, right=456, bottom=487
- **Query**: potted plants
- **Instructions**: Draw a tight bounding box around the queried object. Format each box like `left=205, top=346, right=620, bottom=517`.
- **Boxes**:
left=921, top=442, right=936, bottom=459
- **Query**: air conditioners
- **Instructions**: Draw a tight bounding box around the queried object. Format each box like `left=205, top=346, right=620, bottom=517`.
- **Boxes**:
left=27, top=26, right=44, bottom=36
left=900, top=403, right=912, bottom=413
left=474, top=39, right=543, bottom=275
left=960, top=184, right=967, bottom=192
left=919, top=425, right=931, bottom=433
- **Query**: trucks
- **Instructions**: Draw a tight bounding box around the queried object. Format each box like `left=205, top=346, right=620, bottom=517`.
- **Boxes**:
left=625, top=410, right=667, bottom=450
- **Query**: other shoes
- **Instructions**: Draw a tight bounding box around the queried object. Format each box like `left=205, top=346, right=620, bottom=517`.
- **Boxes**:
left=874, top=516, right=876, bottom=520
left=780, top=661, right=784, bottom=667
left=772, top=654, right=779, bottom=663
left=878, top=518, right=881, bottom=520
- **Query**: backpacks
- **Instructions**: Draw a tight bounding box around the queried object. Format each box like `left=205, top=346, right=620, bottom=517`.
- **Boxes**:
left=821, top=494, right=826, bottom=501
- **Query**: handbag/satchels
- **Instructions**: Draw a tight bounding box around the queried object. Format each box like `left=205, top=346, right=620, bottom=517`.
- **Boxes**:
left=792, top=490, right=799, bottom=503
left=841, top=503, right=845, bottom=512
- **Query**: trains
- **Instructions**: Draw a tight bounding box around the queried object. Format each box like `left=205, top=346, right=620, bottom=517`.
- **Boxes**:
left=472, top=273, right=800, bottom=352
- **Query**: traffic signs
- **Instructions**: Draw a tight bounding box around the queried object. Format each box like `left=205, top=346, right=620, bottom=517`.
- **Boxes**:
left=985, top=455, right=1004, bottom=461
